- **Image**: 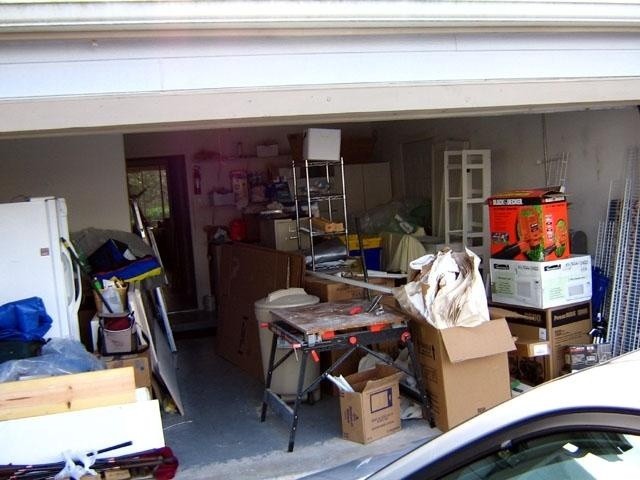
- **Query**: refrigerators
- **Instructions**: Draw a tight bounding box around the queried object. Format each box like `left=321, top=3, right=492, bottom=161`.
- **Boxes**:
left=0, top=196, right=83, bottom=345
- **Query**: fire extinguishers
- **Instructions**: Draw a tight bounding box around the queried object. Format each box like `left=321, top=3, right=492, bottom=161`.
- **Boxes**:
left=194, top=165, right=201, bottom=194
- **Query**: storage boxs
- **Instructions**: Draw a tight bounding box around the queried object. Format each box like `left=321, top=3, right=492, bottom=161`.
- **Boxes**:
left=406, top=308, right=519, bottom=432
left=334, top=363, right=405, bottom=447
left=92, top=347, right=151, bottom=391
left=303, top=129, right=341, bottom=162
left=483, top=186, right=573, bottom=262
left=491, top=302, right=593, bottom=386
left=489, top=253, right=593, bottom=310
left=302, top=273, right=397, bottom=395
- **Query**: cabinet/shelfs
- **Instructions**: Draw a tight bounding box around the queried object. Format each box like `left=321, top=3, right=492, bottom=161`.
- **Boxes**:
left=331, top=162, right=393, bottom=217
left=443, top=150, right=491, bottom=285
left=290, top=157, right=350, bottom=271
